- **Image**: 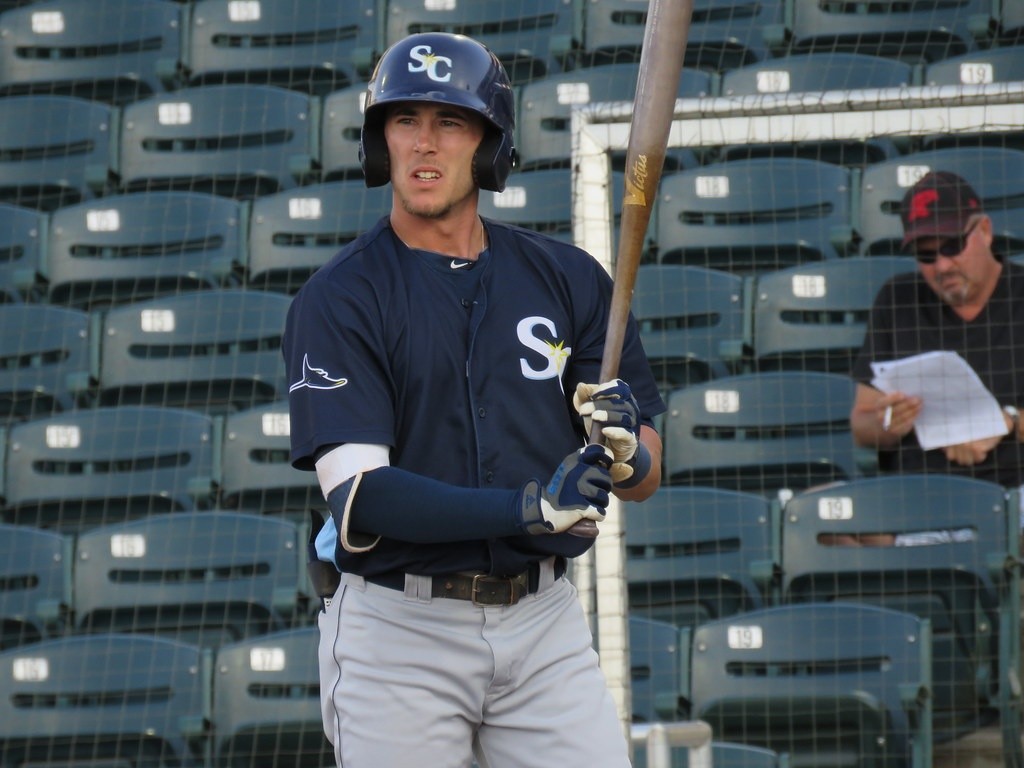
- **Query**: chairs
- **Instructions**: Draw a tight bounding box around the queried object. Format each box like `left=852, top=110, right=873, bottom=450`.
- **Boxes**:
left=0, top=0, right=1024, bottom=767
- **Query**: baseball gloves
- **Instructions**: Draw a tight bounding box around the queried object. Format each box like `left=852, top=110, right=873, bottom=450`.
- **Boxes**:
left=562, top=0, right=696, bottom=540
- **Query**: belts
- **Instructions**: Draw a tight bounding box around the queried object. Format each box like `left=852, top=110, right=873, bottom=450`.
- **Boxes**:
left=366, top=554, right=567, bottom=608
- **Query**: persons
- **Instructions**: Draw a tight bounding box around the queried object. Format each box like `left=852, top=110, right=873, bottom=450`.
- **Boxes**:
left=795, top=169, right=1024, bottom=548
left=282, top=32, right=663, bottom=768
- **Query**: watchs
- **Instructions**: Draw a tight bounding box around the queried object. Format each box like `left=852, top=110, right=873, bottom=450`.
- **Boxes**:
left=1003, top=404, right=1019, bottom=432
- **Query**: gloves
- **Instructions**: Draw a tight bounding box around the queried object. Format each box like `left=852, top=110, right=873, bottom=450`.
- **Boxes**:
left=573, top=379, right=642, bottom=466
left=517, top=442, right=614, bottom=536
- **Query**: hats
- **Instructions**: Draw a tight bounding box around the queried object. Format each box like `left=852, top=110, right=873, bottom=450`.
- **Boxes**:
left=901, top=169, right=984, bottom=249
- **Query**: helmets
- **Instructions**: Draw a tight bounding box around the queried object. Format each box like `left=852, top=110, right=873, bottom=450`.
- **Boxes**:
left=357, top=30, right=517, bottom=192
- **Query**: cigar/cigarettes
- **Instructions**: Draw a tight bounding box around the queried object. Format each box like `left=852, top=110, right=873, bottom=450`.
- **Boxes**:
left=883, top=405, right=893, bottom=430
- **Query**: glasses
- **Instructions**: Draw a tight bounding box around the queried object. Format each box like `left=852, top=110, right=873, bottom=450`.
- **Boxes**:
left=914, top=220, right=978, bottom=263
